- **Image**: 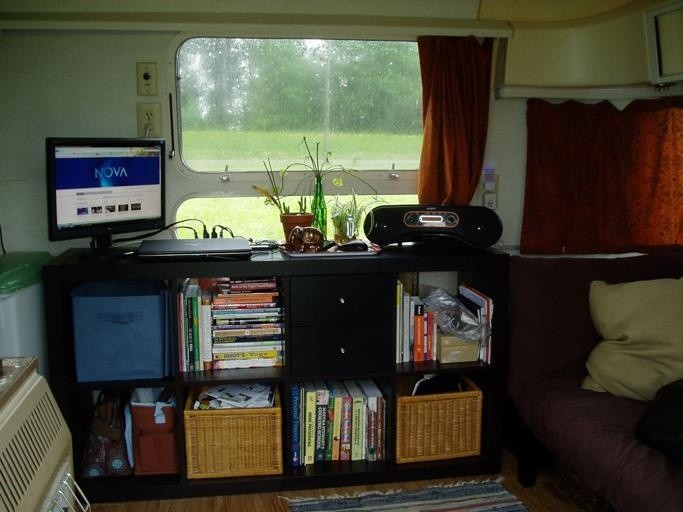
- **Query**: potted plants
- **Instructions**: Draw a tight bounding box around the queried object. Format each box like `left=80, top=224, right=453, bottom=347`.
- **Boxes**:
left=253, top=137, right=389, bottom=250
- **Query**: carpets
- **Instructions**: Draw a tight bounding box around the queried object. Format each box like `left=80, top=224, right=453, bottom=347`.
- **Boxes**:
left=278, top=482, right=526, bottom=511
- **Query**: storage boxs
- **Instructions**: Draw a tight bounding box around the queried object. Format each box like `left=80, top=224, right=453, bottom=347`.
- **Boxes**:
left=436, top=330, right=481, bottom=365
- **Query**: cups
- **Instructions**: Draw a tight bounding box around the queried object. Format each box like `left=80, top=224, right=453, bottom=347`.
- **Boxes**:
left=332, top=213, right=361, bottom=245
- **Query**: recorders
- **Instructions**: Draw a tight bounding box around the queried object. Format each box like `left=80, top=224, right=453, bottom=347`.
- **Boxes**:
left=363, top=205, right=502, bottom=249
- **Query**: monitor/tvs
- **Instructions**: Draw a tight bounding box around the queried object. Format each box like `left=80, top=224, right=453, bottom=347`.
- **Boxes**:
left=45, top=136, right=166, bottom=262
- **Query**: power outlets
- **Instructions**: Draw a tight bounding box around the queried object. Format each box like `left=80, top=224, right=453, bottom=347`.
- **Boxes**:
left=135, top=61, right=159, bottom=96
left=136, top=102, right=161, bottom=138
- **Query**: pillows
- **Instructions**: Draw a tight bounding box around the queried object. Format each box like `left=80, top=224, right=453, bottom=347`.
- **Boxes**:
left=578, top=272, right=682, bottom=401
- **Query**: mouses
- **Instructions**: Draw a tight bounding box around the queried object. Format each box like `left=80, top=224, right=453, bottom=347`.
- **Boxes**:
left=339, top=241, right=367, bottom=253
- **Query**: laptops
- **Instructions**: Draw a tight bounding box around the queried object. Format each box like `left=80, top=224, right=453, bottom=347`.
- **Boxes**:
left=138, top=237, right=252, bottom=262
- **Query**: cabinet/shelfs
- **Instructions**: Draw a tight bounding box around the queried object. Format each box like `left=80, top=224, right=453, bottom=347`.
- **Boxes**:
left=43, top=242, right=512, bottom=504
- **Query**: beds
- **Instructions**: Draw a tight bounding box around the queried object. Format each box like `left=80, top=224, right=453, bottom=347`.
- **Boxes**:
left=513, top=246, right=682, bottom=511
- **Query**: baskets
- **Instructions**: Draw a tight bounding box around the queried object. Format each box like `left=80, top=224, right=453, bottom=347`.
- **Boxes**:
left=181, top=383, right=286, bottom=479
left=390, top=374, right=486, bottom=465
